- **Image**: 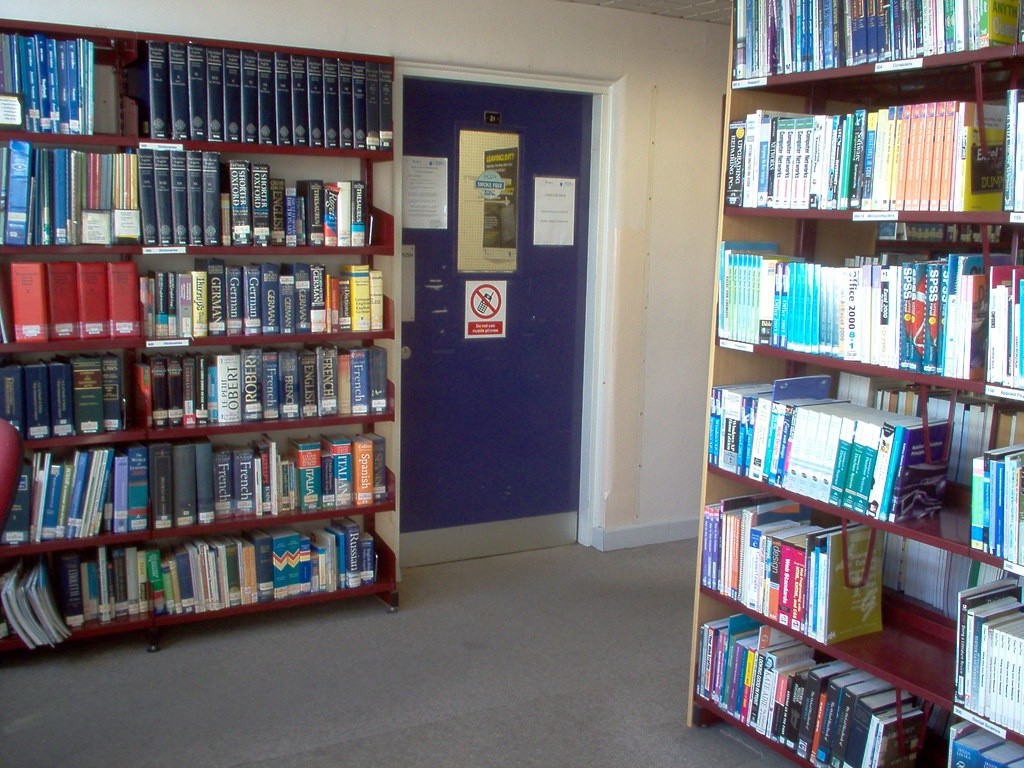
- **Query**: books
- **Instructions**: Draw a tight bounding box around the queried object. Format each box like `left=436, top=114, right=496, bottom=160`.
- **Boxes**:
left=0, top=33, right=394, bottom=650
left=695, top=0, right=1024, bottom=768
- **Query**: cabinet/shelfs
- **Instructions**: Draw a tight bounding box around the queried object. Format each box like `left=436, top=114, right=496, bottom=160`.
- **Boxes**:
left=687, top=0, right=1024, bottom=768
left=0, top=17, right=396, bottom=651
